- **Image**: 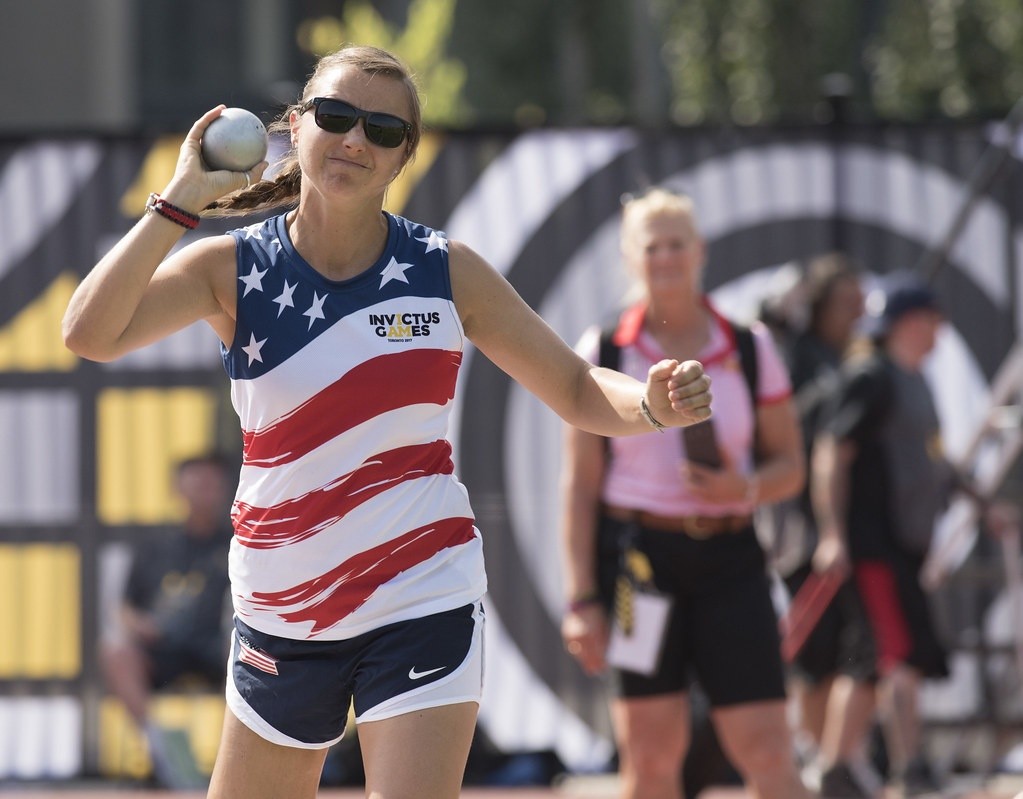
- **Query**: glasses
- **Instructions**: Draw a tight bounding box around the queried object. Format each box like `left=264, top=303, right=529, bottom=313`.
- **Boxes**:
left=300, top=97, right=413, bottom=148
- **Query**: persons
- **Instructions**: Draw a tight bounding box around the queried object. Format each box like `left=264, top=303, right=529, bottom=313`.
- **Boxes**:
left=760, top=254, right=971, bottom=799
left=62, top=46, right=713, bottom=799
left=560, top=189, right=808, bottom=799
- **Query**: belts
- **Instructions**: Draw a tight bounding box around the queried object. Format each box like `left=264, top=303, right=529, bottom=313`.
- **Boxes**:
left=602, top=504, right=751, bottom=540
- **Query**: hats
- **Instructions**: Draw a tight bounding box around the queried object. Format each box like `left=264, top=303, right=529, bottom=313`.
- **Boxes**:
left=868, top=280, right=947, bottom=322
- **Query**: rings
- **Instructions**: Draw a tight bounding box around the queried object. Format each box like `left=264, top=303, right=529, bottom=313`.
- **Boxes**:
left=241, top=170, right=251, bottom=191
left=565, top=640, right=584, bottom=656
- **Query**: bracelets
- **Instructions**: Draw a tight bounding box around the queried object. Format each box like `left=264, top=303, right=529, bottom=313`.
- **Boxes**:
left=145, top=190, right=201, bottom=231
left=640, top=391, right=670, bottom=434
left=564, top=591, right=605, bottom=616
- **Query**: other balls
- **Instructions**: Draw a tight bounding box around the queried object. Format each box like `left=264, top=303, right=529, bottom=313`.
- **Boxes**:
left=202, top=107, right=268, bottom=173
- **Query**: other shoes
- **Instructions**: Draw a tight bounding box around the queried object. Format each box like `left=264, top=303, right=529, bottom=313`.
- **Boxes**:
left=801, top=757, right=885, bottom=799
left=898, top=763, right=982, bottom=799
